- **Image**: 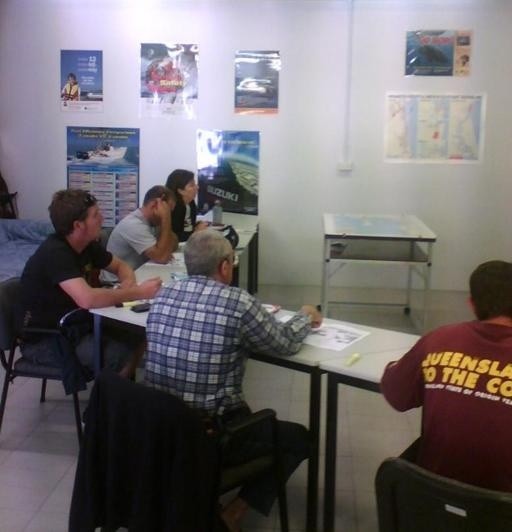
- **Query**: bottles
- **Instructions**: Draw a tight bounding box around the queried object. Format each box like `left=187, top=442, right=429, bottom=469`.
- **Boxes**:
left=213, top=201, right=223, bottom=226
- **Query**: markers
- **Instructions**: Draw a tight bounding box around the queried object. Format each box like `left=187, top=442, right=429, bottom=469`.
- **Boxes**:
left=115, top=301, right=143, bottom=308
left=345, top=353, right=359, bottom=369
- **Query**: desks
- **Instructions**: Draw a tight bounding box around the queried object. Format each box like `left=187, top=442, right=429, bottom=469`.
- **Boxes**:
left=95, top=305, right=375, bottom=531
left=319, top=346, right=413, bottom=532
left=321, top=217, right=436, bottom=336
left=118, top=221, right=258, bottom=296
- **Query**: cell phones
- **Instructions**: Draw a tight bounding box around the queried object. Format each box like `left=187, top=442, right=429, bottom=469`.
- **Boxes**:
left=130, top=302, right=152, bottom=312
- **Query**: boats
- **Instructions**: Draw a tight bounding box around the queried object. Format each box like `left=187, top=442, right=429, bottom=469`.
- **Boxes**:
left=71, top=146, right=128, bottom=162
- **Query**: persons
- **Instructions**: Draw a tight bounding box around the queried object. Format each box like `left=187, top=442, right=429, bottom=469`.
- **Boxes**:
left=381, top=261, right=512, bottom=494
left=16, top=189, right=163, bottom=425
left=143, top=230, right=321, bottom=531
left=100, top=170, right=208, bottom=288
left=60, top=73, right=80, bottom=107
left=102, top=143, right=113, bottom=156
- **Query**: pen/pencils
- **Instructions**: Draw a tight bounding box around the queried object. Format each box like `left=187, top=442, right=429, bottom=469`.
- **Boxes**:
left=151, top=192, right=166, bottom=211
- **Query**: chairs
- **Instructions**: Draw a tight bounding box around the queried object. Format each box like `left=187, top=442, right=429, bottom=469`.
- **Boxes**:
left=0, top=277, right=95, bottom=430
left=376, top=436, right=512, bottom=532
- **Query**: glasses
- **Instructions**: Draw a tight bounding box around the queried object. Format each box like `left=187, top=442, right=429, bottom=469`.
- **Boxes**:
left=82, top=193, right=95, bottom=212
